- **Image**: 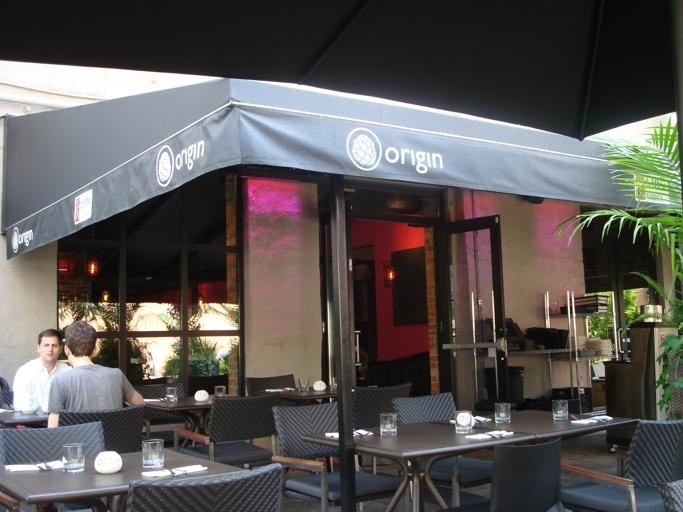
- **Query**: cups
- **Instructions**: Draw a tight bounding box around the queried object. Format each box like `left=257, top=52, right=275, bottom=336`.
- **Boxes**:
left=550, top=399, right=568, bottom=422
left=654, top=304, right=663, bottom=322
left=330, top=378, right=337, bottom=392
left=296, top=377, right=309, bottom=392
left=634, top=303, right=654, bottom=322
left=61, top=443, right=86, bottom=474
left=165, top=387, right=177, bottom=402
left=379, top=412, right=398, bottom=436
left=494, top=402, right=511, bottom=424
left=548, top=300, right=560, bottom=315
left=141, top=440, right=165, bottom=469
left=454, top=411, right=472, bottom=435
left=214, top=385, right=226, bottom=397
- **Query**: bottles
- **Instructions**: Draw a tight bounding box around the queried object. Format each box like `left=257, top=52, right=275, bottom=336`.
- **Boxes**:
left=194, top=390, right=209, bottom=402
left=92, top=450, right=123, bottom=475
left=311, top=379, right=326, bottom=391
left=457, top=413, right=474, bottom=428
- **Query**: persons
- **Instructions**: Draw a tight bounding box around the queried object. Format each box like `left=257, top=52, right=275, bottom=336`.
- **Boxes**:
left=12, top=329, right=71, bottom=429
left=47, top=320, right=144, bottom=512
left=356, top=349, right=375, bottom=386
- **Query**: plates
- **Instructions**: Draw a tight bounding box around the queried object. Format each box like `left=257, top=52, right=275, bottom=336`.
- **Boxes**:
left=15, top=407, right=39, bottom=415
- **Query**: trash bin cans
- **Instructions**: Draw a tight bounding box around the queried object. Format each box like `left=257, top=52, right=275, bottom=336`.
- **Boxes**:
left=483, top=366, right=525, bottom=411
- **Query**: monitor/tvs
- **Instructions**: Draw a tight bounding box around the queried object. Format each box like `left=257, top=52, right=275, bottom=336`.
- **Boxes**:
left=392, top=247, right=427, bottom=324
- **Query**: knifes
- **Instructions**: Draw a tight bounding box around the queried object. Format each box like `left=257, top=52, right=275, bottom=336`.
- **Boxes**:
left=36, top=465, right=46, bottom=473
